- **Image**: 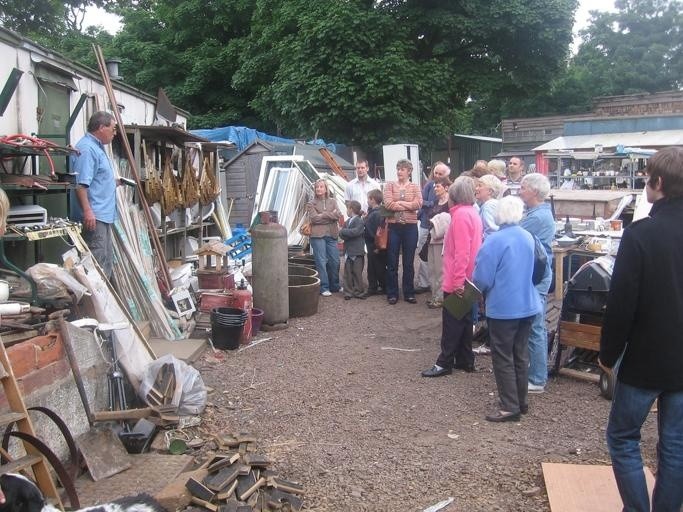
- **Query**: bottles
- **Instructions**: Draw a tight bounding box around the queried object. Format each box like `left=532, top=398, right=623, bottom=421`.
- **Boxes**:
left=564, top=215, right=572, bottom=238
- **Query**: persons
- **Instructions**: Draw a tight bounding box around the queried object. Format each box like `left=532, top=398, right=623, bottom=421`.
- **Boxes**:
left=68, top=111, right=129, bottom=281
left=339, top=200, right=368, bottom=300
left=306, top=178, right=343, bottom=298
left=472, top=195, right=552, bottom=422
left=343, top=156, right=556, bottom=394
left=598, top=146, right=683, bottom=512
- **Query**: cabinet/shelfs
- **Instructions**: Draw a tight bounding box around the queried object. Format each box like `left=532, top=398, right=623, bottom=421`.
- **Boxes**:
left=108, top=128, right=218, bottom=273
left=0, top=133, right=70, bottom=324
left=542, top=151, right=650, bottom=191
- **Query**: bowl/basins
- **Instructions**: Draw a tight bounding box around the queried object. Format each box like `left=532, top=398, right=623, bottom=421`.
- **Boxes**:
left=555, top=239, right=576, bottom=247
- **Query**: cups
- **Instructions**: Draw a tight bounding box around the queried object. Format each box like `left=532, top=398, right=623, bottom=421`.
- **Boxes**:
left=576, top=170, right=618, bottom=177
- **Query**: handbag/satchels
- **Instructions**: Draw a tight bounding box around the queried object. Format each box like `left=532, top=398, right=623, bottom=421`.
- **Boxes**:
left=375, top=226, right=387, bottom=253
left=380, top=202, right=395, bottom=218
left=533, top=239, right=547, bottom=286
left=419, top=233, right=431, bottom=262
left=299, top=219, right=313, bottom=236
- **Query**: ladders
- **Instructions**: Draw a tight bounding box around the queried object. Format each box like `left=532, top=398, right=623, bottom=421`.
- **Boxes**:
left=0, top=337, right=66, bottom=512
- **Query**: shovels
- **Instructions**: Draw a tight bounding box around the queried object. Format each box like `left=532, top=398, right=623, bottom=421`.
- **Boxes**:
left=48, top=309, right=133, bottom=483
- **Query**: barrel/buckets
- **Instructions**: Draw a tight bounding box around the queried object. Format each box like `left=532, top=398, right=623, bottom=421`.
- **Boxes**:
left=251, top=307, right=264, bottom=336
left=211, top=307, right=248, bottom=350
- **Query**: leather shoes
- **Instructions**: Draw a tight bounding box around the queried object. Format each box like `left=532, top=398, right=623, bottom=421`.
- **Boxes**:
left=485, top=405, right=528, bottom=422
left=452, top=361, right=474, bottom=372
left=422, top=365, right=451, bottom=378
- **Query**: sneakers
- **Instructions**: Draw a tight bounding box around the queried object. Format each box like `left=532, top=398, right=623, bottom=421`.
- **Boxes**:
left=321, top=286, right=343, bottom=297
left=388, top=297, right=398, bottom=304
left=344, top=286, right=384, bottom=299
left=472, top=343, right=492, bottom=355
left=528, top=380, right=545, bottom=394
left=415, top=285, right=430, bottom=294
left=405, top=296, right=417, bottom=303
left=426, top=297, right=444, bottom=308
